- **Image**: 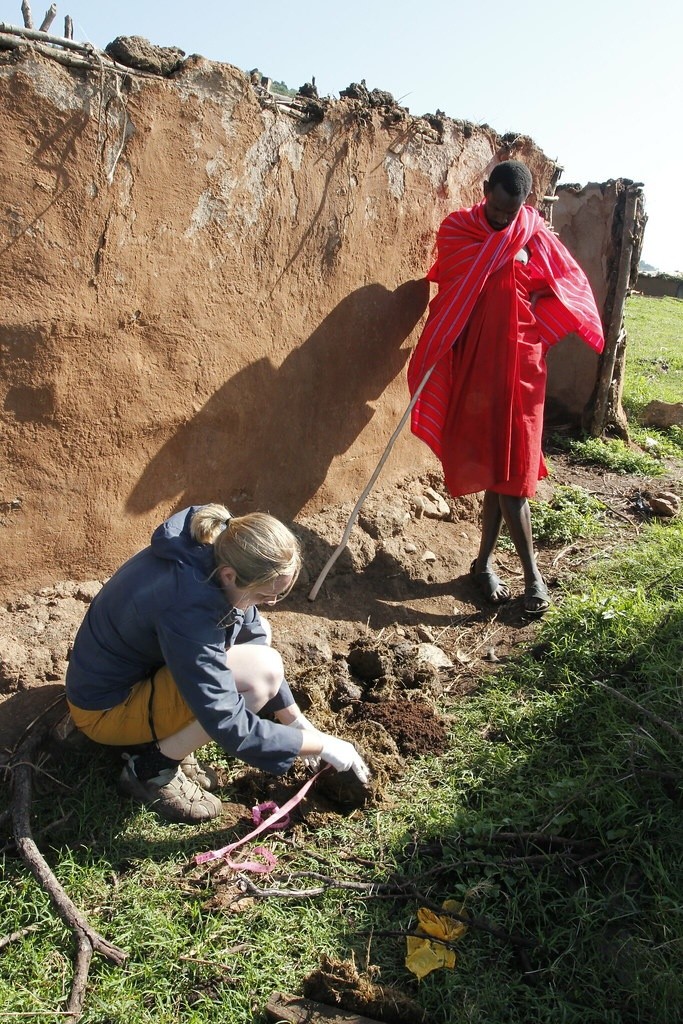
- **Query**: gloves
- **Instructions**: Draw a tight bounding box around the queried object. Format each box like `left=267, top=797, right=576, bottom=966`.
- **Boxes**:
left=286, top=713, right=322, bottom=773
left=317, top=732, right=371, bottom=783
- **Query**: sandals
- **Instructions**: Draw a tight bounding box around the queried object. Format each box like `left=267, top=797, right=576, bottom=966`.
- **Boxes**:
left=470, top=559, right=511, bottom=604
left=524, top=576, right=551, bottom=615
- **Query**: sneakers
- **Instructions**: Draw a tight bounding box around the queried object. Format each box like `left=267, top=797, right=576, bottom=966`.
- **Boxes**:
left=179, top=752, right=219, bottom=791
left=119, top=751, right=222, bottom=823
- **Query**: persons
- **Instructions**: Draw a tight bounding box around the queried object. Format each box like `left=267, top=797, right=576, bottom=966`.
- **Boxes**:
left=407, top=160, right=604, bottom=614
left=65, top=503, right=368, bottom=826
